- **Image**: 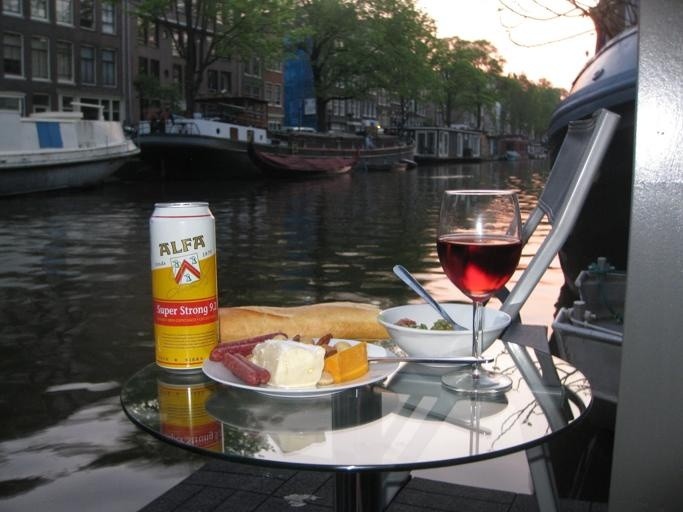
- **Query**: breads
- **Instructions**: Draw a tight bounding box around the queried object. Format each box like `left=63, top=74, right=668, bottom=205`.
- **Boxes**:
left=250, top=337, right=325, bottom=387
left=217, top=301, right=390, bottom=340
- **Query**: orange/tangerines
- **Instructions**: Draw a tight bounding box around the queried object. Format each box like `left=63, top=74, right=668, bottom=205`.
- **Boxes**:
left=325, top=341, right=369, bottom=383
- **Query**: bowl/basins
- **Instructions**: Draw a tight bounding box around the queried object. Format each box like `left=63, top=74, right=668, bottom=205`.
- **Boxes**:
left=373, top=299, right=513, bottom=371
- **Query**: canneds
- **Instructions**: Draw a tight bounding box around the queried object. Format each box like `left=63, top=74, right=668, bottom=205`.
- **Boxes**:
left=156, top=374, right=224, bottom=455
left=149, top=202, right=221, bottom=374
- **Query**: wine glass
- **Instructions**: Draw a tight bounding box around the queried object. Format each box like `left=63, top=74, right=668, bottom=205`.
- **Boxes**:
left=432, top=187, right=523, bottom=397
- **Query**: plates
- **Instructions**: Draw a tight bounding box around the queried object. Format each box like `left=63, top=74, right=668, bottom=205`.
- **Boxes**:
left=198, top=336, right=403, bottom=400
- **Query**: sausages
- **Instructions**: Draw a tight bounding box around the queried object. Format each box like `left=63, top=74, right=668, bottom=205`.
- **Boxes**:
left=209, top=332, right=288, bottom=386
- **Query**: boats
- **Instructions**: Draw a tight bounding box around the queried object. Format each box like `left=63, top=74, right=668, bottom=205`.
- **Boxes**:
left=400, top=125, right=482, bottom=163
left=134, top=92, right=417, bottom=180
left=0, top=99, right=142, bottom=199
